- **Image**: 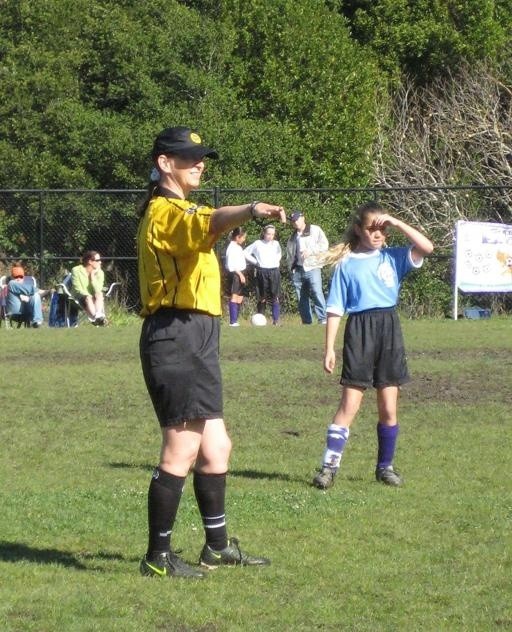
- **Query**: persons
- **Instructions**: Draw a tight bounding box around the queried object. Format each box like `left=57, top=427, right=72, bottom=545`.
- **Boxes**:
left=224, top=226, right=248, bottom=328
left=243, top=224, right=282, bottom=328
left=70, top=249, right=108, bottom=328
left=287, top=211, right=329, bottom=324
left=0, top=265, right=56, bottom=329
left=312, top=202, right=433, bottom=490
left=135, top=127, right=287, bottom=579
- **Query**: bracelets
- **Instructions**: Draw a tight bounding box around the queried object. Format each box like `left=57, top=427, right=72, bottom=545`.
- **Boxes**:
left=248, top=200, right=266, bottom=224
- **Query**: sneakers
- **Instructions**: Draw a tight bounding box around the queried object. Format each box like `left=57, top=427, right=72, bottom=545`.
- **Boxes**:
left=314, top=466, right=336, bottom=490
left=139, top=550, right=208, bottom=579
left=199, top=537, right=270, bottom=571
left=375, top=466, right=402, bottom=486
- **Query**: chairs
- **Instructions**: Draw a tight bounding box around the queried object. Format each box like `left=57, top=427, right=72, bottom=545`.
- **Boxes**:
left=0, top=273, right=41, bottom=329
left=57, top=273, right=120, bottom=328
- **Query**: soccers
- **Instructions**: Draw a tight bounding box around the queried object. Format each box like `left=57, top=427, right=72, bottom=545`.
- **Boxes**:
left=251, top=313, right=267, bottom=327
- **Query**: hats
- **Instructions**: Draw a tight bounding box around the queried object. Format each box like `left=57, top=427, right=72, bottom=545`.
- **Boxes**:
left=291, top=212, right=301, bottom=220
left=12, top=267, right=25, bottom=277
left=154, top=127, right=219, bottom=161
